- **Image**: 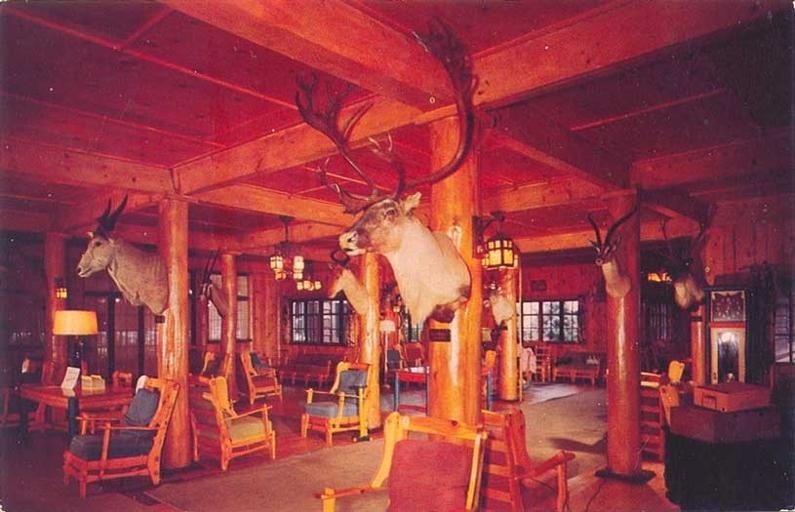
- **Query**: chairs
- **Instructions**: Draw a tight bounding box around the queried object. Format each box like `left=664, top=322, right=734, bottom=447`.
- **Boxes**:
left=605, top=372, right=667, bottom=461
left=382, top=341, right=550, bottom=401
left=188, top=364, right=280, bottom=473
left=62, top=370, right=181, bottom=498
left=1, top=341, right=346, bottom=449
left=298, top=361, right=375, bottom=446
left=479, top=404, right=579, bottom=510
left=550, top=350, right=608, bottom=386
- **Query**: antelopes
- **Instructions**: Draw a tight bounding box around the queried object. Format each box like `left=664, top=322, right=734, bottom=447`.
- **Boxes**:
left=587, top=202, right=639, bottom=298
left=195, top=247, right=229, bottom=319
left=75, top=192, right=169, bottom=316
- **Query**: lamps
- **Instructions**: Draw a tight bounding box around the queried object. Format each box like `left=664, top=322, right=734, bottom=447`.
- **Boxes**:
left=52, top=310, right=99, bottom=393
left=269, top=216, right=322, bottom=293
left=481, top=215, right=514, bottom=268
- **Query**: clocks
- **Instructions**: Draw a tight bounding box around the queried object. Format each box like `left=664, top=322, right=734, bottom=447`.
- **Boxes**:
left=702, top=283, right=764, bottom=383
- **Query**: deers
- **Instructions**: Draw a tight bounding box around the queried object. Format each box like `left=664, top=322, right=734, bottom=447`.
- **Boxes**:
left=481, top=265, right=516, bottom=326
left=323, top=247, right=369, bottom=320
left=654, top=206, right=711, bottom=310
left=292, top=13, right=472, bottom=331
